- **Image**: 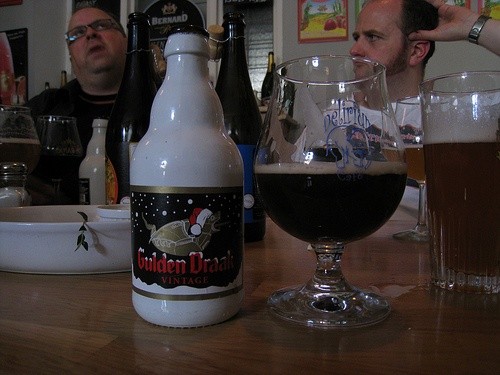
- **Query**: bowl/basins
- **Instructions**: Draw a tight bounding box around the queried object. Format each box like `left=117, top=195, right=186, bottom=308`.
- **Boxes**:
left=0, top=205, right=131, bottom=275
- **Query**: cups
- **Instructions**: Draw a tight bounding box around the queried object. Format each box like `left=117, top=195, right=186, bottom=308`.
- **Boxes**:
left=0, top=105, right=40, bottom=190
left=97, top=205, right=130, bottom=220
left=419, top=71, right=500, bottom=294
left=34, top=115, right=83, bottom=205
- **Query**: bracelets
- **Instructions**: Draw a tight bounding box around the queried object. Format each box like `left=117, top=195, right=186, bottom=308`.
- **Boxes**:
left=468, top=15, right=490, bottom=44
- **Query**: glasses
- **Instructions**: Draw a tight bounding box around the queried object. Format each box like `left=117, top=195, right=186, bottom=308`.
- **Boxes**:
left=65, top=18, right=125, bottom=41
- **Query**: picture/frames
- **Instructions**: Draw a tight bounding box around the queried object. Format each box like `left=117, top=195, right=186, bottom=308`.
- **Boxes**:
left=0, top=27, right=30, bottom=105
left=297, top=0, right=349, bottom=44
left=478, top=0, right=500, bottom=19
left=443, top=0, right=471, bottom=12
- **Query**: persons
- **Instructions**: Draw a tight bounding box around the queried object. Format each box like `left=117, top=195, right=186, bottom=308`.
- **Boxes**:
left=0, top=5, right=162, bottom=205
left=287, top=0, right=500, bottom=210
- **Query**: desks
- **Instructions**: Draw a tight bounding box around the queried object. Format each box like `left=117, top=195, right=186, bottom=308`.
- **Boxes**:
left=0, top=198, right=500, bottom=375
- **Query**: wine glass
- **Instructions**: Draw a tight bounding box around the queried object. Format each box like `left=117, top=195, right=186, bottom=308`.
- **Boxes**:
left=254, top=54, right=407, bottom=331
left=393, top=96, right=431, bottom=241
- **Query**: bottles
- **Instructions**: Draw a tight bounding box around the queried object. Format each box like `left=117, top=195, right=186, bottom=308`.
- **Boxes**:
left=0, top=162, right=27, bottom=207
left=130, top=24, right=243, bottom=328
left=79, top=116, right=108, bottom=205
left=261, top=51, right=275, bottom=113
left=60, top=70, right=67, bottom=88
left=213, top=12, right=266, bottom=243
left=44, top=82, right=50, bottom=90
left=105, top=13, right=162, bottom=203
